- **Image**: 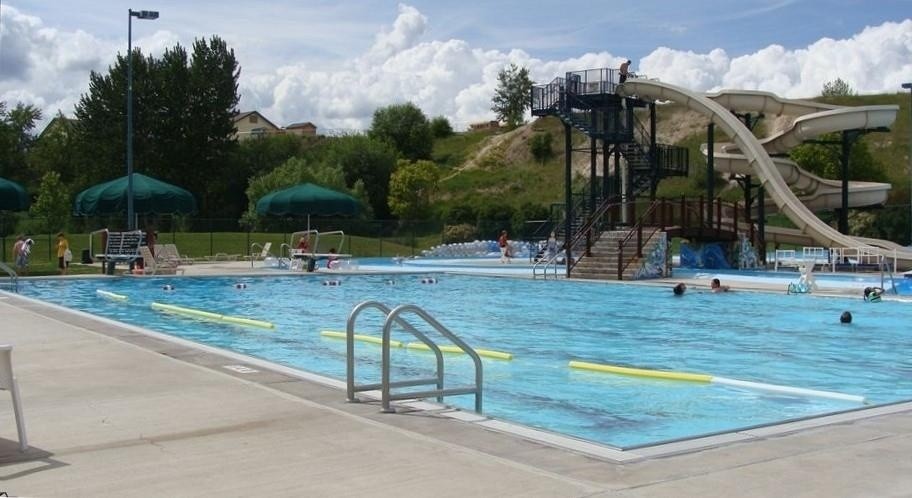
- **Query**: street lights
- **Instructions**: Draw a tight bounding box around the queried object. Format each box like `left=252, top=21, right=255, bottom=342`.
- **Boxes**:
left=122, top=6, right=163, bottom=269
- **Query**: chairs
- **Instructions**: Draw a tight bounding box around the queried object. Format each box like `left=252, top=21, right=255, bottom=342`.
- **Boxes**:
left=243, top=240, right=273, bottom=273
left=136, top=242, right=195, bottom=276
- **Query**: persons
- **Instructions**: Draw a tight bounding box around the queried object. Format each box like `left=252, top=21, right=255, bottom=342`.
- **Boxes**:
left=673, top=282, right=687, bottom=296
left=55, top=231, right=69, bottom=274
left=547, top=231, right=558, bottom=265
left=498, top=229, right=511, bottom=263
left=840, top=309, right=853, bottom=322
left=12, top=234, right=24, bottom=261
left=710, top=277, right=729, bottom=292
left=619, top=59, right=632, bottom=83
left=145, top=226, right=156, bottom=259
left=15, top=238, right=35, bottom=271
left=327, top=247, right=339, bottom=269
left=293, top=237, right=309, bottom=259
left=863, top=286, right=885, bottom=302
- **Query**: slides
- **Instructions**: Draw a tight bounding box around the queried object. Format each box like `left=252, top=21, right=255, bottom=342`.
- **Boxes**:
left=615, top=77, right=912, bottom=269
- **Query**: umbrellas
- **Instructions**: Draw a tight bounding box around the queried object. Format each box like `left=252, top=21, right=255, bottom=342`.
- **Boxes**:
left=71, top=172, right=199, bottom=230
left=256, top=181, right=355, bottom=239
left=0, top=177, right=32, bottom=211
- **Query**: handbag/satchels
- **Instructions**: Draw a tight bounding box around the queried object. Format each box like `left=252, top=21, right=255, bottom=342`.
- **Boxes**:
left=64, top=249, right=72, bottom=262
